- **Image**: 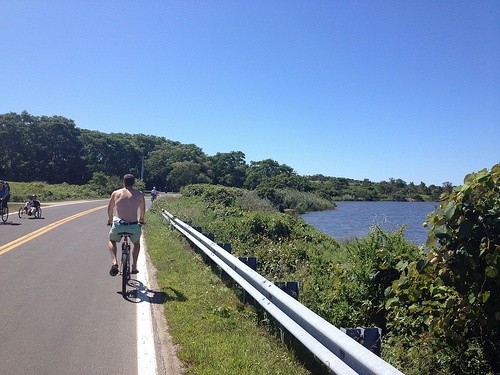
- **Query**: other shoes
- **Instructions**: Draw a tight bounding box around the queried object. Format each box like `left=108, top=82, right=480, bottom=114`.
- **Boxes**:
left=110, top=264, right=118, bottom=276
left=132, top=270, right=139, bottom=274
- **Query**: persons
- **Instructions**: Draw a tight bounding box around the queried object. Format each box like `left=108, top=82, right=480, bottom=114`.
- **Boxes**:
left=0, top=180, right=10, bottom=215
left=25, top=194, right=40, bottom=216
left=107, top=174, right=146, bottom=277
left=151, top=187, right=158, bottom=200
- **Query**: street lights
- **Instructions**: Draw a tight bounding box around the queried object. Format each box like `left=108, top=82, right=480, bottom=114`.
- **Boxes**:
left=141, top=155, right=147, bottom=192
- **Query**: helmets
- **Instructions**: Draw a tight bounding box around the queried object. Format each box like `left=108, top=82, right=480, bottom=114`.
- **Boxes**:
left=0, top=180, right=4, bottom=184
left=31, top=194, right=37, bottom=200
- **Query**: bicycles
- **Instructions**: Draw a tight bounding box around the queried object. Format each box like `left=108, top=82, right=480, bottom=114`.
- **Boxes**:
left=0, top=198, right=9, bottom=222
left=106, top=222, right=147, bottom=298
left=151, top=193, right=159, bottom=203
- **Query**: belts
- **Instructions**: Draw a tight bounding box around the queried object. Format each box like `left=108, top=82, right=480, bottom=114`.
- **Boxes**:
left=120, top=221, right=138, bottom=225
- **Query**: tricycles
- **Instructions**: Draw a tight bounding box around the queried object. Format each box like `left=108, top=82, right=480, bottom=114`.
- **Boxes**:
left=18, top=200, right=42, bottom=219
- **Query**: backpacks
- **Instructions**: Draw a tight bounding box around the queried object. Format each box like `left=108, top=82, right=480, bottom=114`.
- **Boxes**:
left=4, top=182, right=10, bottom=191
